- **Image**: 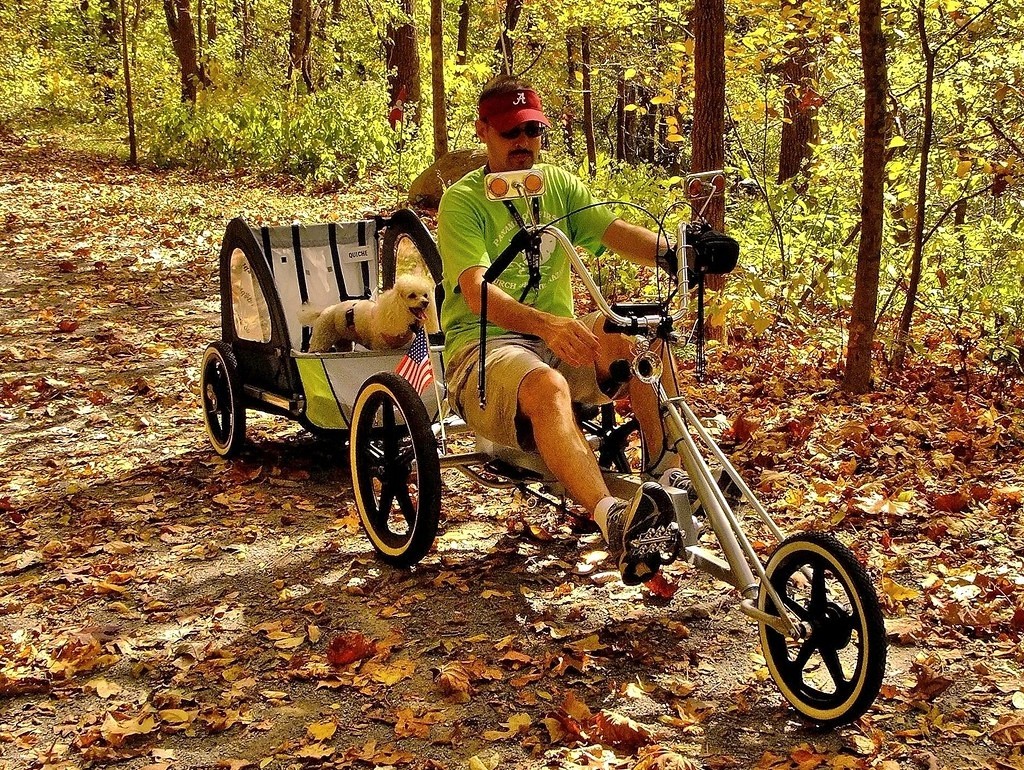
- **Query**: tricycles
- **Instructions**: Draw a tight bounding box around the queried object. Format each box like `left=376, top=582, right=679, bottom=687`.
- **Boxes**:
left=345, top=169, right=882, bottom=727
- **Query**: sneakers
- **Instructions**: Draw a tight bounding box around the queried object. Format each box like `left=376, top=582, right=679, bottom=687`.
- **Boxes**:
left=604, top=482, right=674, bottom=586
left=659, top=466, right=743, bottom=523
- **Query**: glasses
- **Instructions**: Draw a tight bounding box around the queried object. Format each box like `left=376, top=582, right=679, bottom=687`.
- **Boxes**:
left=482, top=123, right=544, bottom=139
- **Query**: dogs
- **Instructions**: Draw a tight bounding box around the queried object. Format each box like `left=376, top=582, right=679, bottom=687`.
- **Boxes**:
left=292, top=273, right=439, bottom=353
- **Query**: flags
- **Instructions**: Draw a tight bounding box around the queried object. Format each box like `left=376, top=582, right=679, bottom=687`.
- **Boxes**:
left=395, top=324, right=434, bottom=397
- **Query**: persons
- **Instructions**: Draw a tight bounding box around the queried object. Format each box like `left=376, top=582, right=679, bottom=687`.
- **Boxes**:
left=437, top=76, right=743, bottom=586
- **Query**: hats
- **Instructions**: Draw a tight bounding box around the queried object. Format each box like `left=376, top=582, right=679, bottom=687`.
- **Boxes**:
left=479, top=89, right=552, bottom=133
